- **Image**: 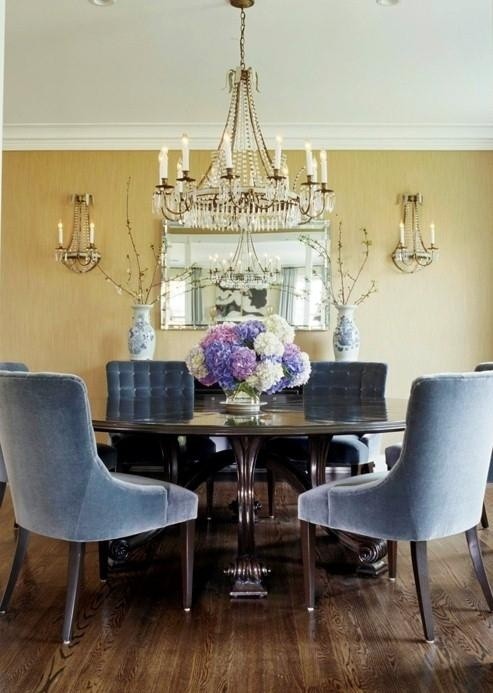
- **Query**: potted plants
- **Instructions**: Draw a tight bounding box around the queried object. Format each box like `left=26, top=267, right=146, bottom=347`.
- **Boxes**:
left=332, top=305, right=361, bottom=362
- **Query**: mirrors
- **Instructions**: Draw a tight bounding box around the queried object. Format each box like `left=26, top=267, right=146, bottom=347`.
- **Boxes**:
left=161, top=230, right=336, bottom=331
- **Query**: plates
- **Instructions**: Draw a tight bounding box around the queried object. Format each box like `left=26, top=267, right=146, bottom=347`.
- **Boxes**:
left=218, top=402, right=269, bottom=410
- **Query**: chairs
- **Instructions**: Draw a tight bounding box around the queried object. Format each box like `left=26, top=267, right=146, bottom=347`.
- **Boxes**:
left=0, top=361, right=115, bottom=505
left=106, top=359, right=217, bottom=511
left=263, top=361, right=390, bottom=520
left=295, top=368, right=493, bottom=643
left=381, top=361, right=493, bottom=528
left=0, top=368, right=203, bottom=643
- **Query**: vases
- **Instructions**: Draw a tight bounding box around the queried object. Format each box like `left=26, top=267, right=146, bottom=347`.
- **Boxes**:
left=219, top=390, right=270, bottom=414
left=127, top=306, right=156, bottom=361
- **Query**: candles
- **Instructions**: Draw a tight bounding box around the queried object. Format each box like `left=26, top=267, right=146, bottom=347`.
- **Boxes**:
left=179, top=134, right=192, bottom=169
left=273, top=122, right=283, bottom=172
left=216, top=130, right=239, bottom=174
left=174, top=159, right=183, bottom=193
left=398, top=220, right=408, bottom=247
left=88, top=221, right=97, bottom=245
left=303, top=136, right=315, bottom=177
left=430, top=220, right=439, bottom=246
left=320, top=144, right=335, bottom=185
left=205, top=250, right=281, bottom=278
left=55, top=216, right=66, bottom=245
left=156, top=143, right=171, bottom=182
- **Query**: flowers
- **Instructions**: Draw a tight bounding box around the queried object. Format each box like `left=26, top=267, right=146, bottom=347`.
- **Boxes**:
left=299, top=220, right=379, bottom=305
left=186, top=314, right=311, bottom=397
left=89, top=176, right=196, bottom=306
